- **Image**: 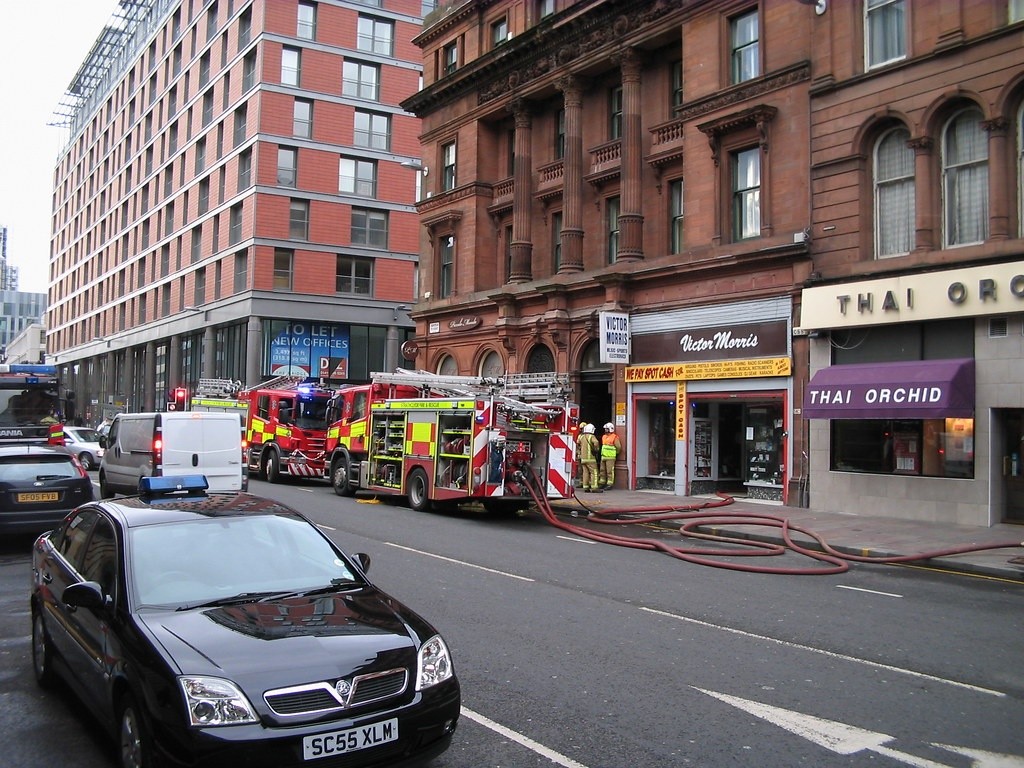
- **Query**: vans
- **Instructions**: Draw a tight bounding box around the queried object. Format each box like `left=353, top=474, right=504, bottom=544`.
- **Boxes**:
left=98, top=412, right=248, bottom=499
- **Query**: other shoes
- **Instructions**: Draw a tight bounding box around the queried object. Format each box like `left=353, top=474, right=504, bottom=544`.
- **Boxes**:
left=604, top=486, right=611, bottom=490
left=598, top=485, right=603, bottom=489
left=593, top=488, right=603, bottom=493
left=584, top=489, right=591, bottom=492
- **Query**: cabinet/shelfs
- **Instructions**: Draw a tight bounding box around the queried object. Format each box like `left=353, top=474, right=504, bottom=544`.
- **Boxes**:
left=649, top=403, right=674, bottom=476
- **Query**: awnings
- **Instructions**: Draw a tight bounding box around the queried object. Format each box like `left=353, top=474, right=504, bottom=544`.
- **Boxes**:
left=803, top=357, right=976, bottom=419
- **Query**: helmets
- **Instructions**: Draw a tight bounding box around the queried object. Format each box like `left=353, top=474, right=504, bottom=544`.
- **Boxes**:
left=579, top=422, right=587, bottom=432
left=603, top=423, right=614, bottom=433
left=583, top=423, right=596, bottom=434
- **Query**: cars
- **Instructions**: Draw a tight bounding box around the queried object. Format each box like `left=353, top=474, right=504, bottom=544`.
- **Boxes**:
left=32, top=473, right=461, bottom=768
left=0, top=444, right=98, bottom=536
left=64, top=426, right=106, bottom=470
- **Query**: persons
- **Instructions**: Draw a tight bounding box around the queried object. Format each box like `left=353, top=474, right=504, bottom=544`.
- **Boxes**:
left=599, top=423, right=622, bottom=490
left=580, top=423, right=603, bottom=493
left=576, top=422, right=587, bottom=488
left=74, top=414, right=83, bottom=427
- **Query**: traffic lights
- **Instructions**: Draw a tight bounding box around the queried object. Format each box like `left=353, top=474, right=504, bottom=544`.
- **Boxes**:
left=175, top=388, right=186, bottom=412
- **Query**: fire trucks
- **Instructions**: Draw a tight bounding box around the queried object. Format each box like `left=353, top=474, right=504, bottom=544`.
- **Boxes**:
left=191, top=373, right=337, bottom=484
left=324, top=368, right=580, bottom=514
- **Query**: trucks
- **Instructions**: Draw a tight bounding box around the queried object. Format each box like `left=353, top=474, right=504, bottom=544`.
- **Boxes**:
left=0, top=363, right=76, bottom=447
